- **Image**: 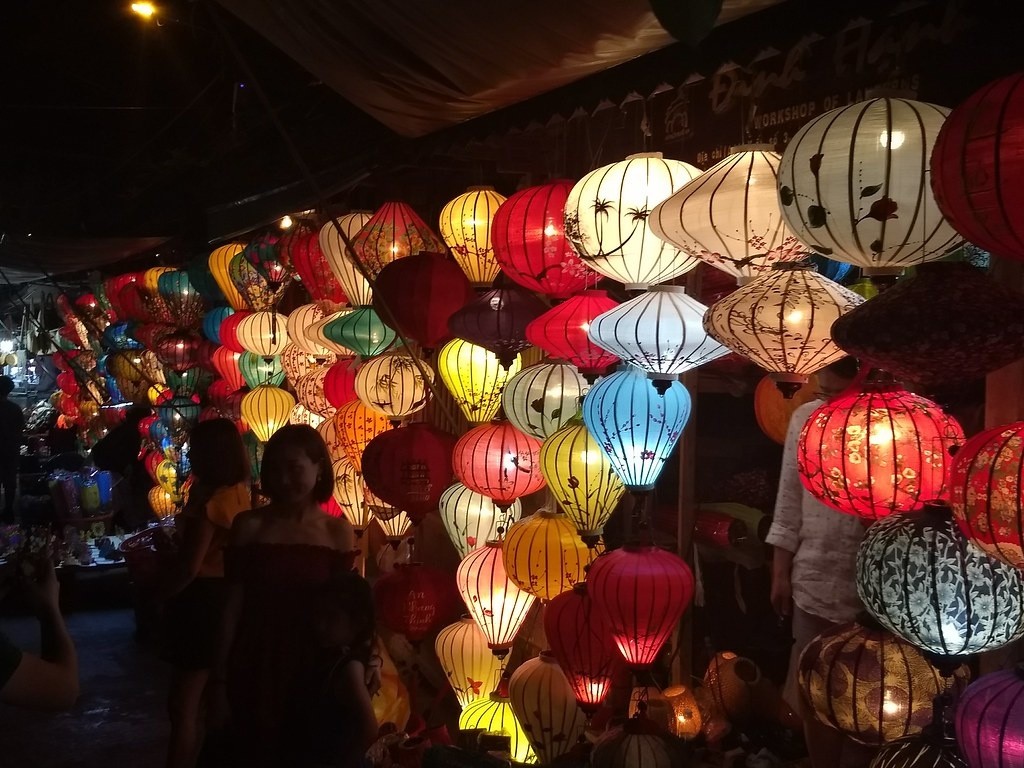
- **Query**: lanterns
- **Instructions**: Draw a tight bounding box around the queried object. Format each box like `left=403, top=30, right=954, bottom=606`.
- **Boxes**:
left=437, top=69, right=1024, bottom=768
left=50, top=184, right=478, bottom=648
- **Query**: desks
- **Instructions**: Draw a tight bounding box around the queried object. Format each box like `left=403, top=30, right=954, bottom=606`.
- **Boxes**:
left=58, top=506, right=115, bottom=539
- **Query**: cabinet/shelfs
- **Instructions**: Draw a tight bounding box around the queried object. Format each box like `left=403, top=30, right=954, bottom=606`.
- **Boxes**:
left=621, top=265, right=780, bottom=673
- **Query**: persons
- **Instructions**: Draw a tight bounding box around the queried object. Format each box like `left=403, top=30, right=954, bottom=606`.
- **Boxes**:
left=0, top=376, right=25, bottom=524
left=0, top=560, right=78, bottom=714
left=764, top=355, right=876, bottom=768
left=165, top=419, right=380, bottom=768
